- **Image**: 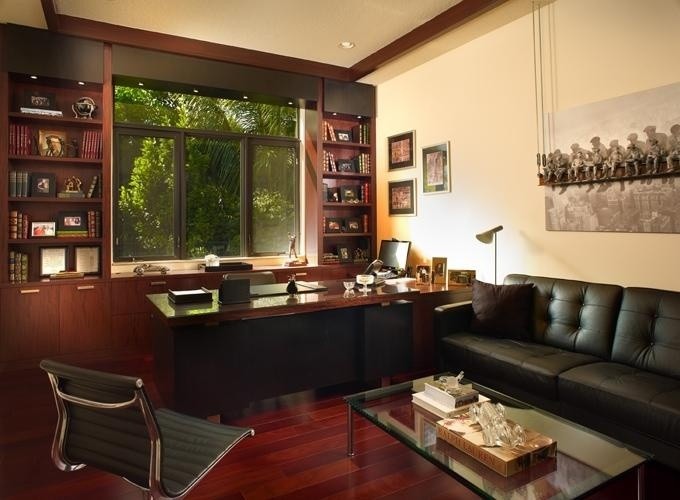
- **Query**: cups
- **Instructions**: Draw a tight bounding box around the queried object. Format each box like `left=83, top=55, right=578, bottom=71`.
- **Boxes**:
left=343, top=291, right=356, bottom=299
left=205, top=254, right=219, bottom=267
left=344, top=282, right=355, bottom=290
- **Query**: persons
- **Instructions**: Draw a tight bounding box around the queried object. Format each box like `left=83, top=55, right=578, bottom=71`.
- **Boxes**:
left=543, top=124, right=680, bottom=196
left=288, top=232, right=297, bottom=259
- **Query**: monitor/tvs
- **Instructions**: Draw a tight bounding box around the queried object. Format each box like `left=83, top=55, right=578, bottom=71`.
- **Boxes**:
left=377, top=239, right=411, bottom=272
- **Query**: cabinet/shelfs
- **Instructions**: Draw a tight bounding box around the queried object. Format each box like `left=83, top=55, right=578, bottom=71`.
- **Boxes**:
left=2, top=24, right=111, bottom=282
left=111, top=279, right=220, bottom=349
left=305, top=77, right=378, bottom=265
left=1, top=283, right=111, bottom=361
left=277, top=266, right=363, bottom=282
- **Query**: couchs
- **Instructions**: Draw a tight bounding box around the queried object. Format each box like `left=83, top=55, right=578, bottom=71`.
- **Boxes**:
left=435, top=275, right=680, bottom=457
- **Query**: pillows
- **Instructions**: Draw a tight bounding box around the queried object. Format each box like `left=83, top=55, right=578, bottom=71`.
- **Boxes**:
left=467, top=279, right=533, bottom=334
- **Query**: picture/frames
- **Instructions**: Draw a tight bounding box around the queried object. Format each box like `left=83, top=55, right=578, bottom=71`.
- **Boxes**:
left=58, top=209, right=87, bottom=229
left=338, top=158, right=355, bottom=172
left=420, top=141, right=451, bottom=196
left=340, top=185, right=357, bottom=201
left=325, top=218, right=341, bottom=232
left=39, top=129, right=67, bottom=157
left=386, top=130, right=416, bottom=172
left=25, top=88, right=55, bottom=109
left=30, top=171, right=55, bottom=195
left=336, top=243, right=353, bottom=264
left=387, top=178, right=417, bottom=216
left=431, top=257, right=447, bottom=285
left=335, top=129, right=353, bottom=142
left=448, top=269, right=475, bottom=284
left=344, top=217, right=363, bottom=233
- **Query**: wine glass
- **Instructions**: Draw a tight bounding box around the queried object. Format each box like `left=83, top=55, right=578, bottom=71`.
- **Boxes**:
left=357, top=275, right=374, bottom=292
left=359, top=293, right=370, bottom=298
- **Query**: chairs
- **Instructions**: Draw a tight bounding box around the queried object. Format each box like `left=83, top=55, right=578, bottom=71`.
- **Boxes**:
left=39, top=357, right=256, bottom=500
left=224, top=271, right=277, bottom=287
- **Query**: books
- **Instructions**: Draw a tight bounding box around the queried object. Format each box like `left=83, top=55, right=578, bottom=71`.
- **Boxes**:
left=321, top=120, right=371, bottom=265
left=8, top=95, right=103, bottom=286
left=411, top=379, right=491, bottom=419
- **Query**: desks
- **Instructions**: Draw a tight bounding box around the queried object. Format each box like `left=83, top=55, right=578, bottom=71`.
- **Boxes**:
left=145, top=277, right=472, bottom=424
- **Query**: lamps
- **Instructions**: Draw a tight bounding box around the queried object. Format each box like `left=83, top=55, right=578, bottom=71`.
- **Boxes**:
left=473, top=225, right=503, bottom=287
